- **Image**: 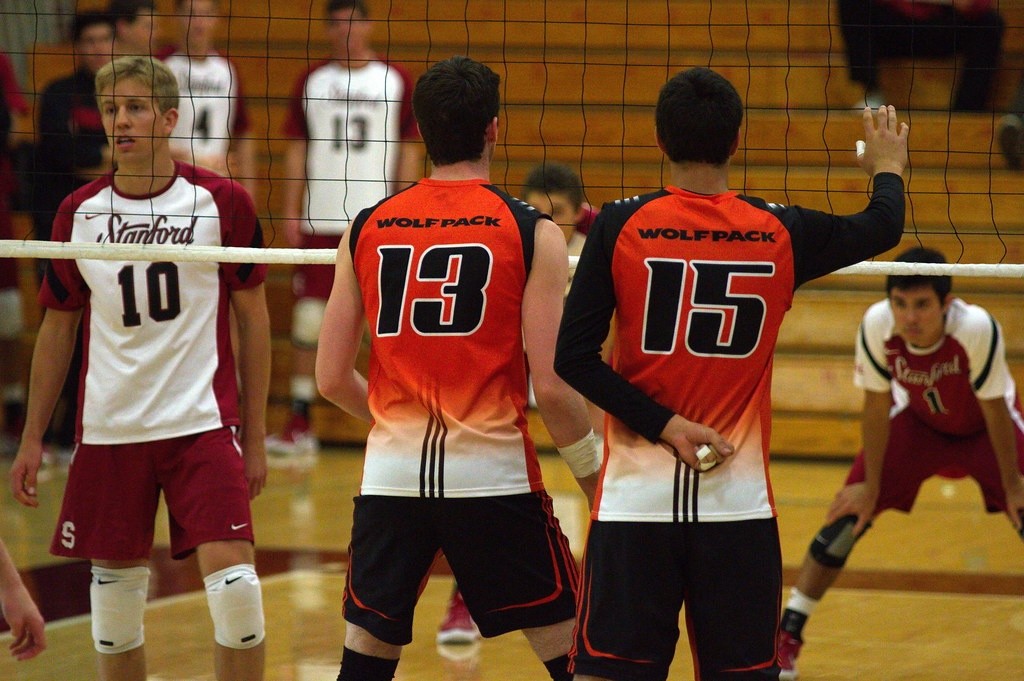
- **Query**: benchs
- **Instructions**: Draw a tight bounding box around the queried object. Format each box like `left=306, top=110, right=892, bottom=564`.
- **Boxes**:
left=0, top=0, right=1024, bottom=459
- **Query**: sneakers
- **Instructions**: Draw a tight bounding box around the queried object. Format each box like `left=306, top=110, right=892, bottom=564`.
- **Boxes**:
left=775, top=621, right=803, bottom=679
left=264, top=413, right=317, bottom=452
left=437, top=583, right=479, bottom=645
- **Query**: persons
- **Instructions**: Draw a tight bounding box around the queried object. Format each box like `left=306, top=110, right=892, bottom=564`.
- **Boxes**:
left=32, top=12, right=118, bottom=488
left=1, top=539, right=46, bottom=660
left=552, top=66, right=907, bottom=681
left=776, top=246, right=1024, bottom=679
left=67, top=0, right=191, bottom=188
left=149, top=1, right=254, bottom=437
left=267, top=0, right=423, bottom=459
left=836, top=0, right=1007, bottom=114
left=313, top=55, right=600, bottom=681
left=11, top=57, right=276, bottom=681
left=432, top=162, right=600, bottom=644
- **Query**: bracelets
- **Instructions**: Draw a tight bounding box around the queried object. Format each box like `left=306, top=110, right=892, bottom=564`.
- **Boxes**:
left=555, top=429, right=600, bottom=480
